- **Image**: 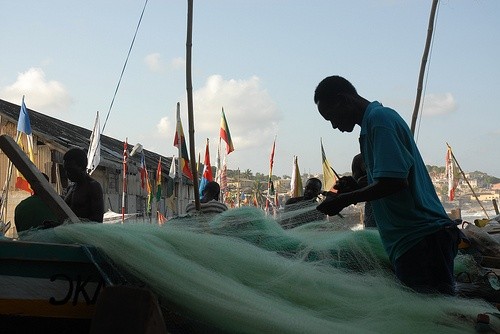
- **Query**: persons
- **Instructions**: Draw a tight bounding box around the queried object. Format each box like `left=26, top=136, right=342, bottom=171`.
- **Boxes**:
left=185, top=182, right=228, bottom=214
left=63, top=147, right=103, bottom=222
left=15, top=172, right=69, bottom=236
left=313, top=75, right=460, bottom=296
left=281, top=178, right=325, bottom=230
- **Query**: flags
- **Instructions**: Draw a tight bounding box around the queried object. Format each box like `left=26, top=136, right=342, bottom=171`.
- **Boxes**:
left=199, top=141, right=212, bottom=201
left=88, top=121, right=101, bottom=175
left=153, top=164, right=161, bottom=213
left=444, top=150, right=459, bottom=200
left=321, top=144, right=336, bottom=193
left=174, top=110, right=193, bottom=181
left=143, top=170, right=152, bottom=223
left=16, top=102, right=31, bottom=135
left=220, top=111, right=235, bottom=155
left=211, top=145, right=276, bottom=210
left=291, top=164, right=303, bottom=197
